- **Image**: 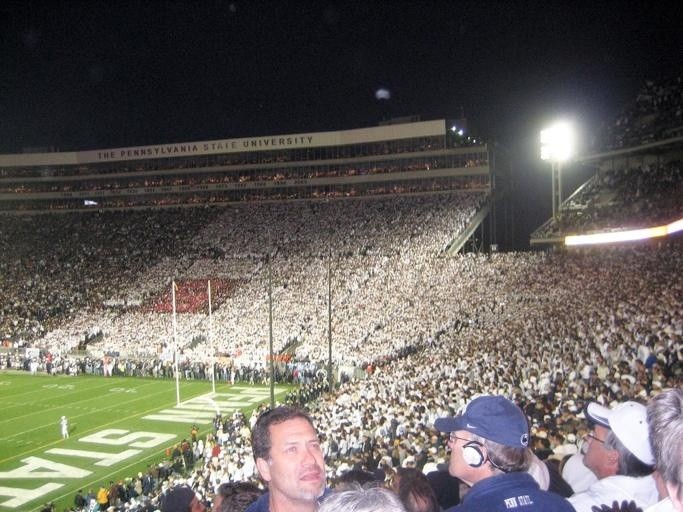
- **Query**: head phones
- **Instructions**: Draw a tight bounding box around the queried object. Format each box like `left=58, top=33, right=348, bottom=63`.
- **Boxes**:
left=461, top=441, right=512, bottom=474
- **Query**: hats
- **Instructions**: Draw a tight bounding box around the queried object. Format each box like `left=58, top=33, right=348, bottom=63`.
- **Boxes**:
left=159, top=487, right=196, bottom=512
left=432, top=395, right=530, bottom=450
left=584, top=400, right=657, bottom=469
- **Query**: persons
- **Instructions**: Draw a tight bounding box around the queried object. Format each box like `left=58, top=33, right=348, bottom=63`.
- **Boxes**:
left=59, top=415, right=70, bottom=441
left=529, top=68, right=683, bottom=248
left=74, top=250, right=682, bottom=510
left=72, top=184, right=491, bottom=249
left=0, top=164, right=74, bottom=377
left=40, top=499, right=56, bottom=512
